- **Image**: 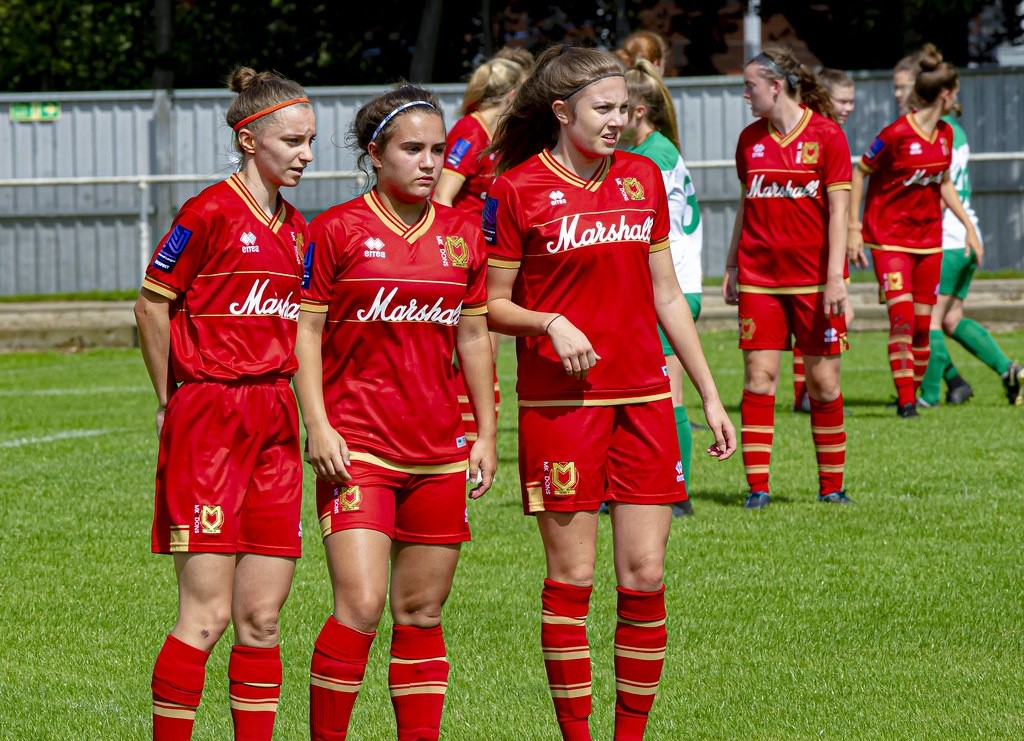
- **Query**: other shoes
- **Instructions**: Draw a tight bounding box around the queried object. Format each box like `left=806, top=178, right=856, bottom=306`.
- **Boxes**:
left=945, top=384, right=974, bottom=406
left=894, top=398, right=918, bottom=418
left=744, top=491, right=769, bottom=508
left=1001, top=362, right=1024, bottom=407
left=793, top=395, right=852, bottom=416
left=817, top=489, right=856, bottom=505
left=672, top=495, right=694, bottom=517
left=915, top=396, right=939, bottom=409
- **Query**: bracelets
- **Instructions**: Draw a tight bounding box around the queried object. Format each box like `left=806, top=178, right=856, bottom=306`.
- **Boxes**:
left=848, top=222, right=863, bottom=232
left=725, top=265, right=737, bottom=268
left=545, top=314, right=563, bottom=334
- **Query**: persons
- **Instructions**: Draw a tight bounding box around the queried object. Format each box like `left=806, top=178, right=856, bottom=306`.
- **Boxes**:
left=427, top=29, right=702, bottom=519
left=132, top=68, right=316, bottom=741
left=848, top=44, right=1023, bottom=418
left=294, top=87, right=498, bottom=741
left=482, top=43, right=737, bottom=741
left=721, top=48, right=857, bottom=510
